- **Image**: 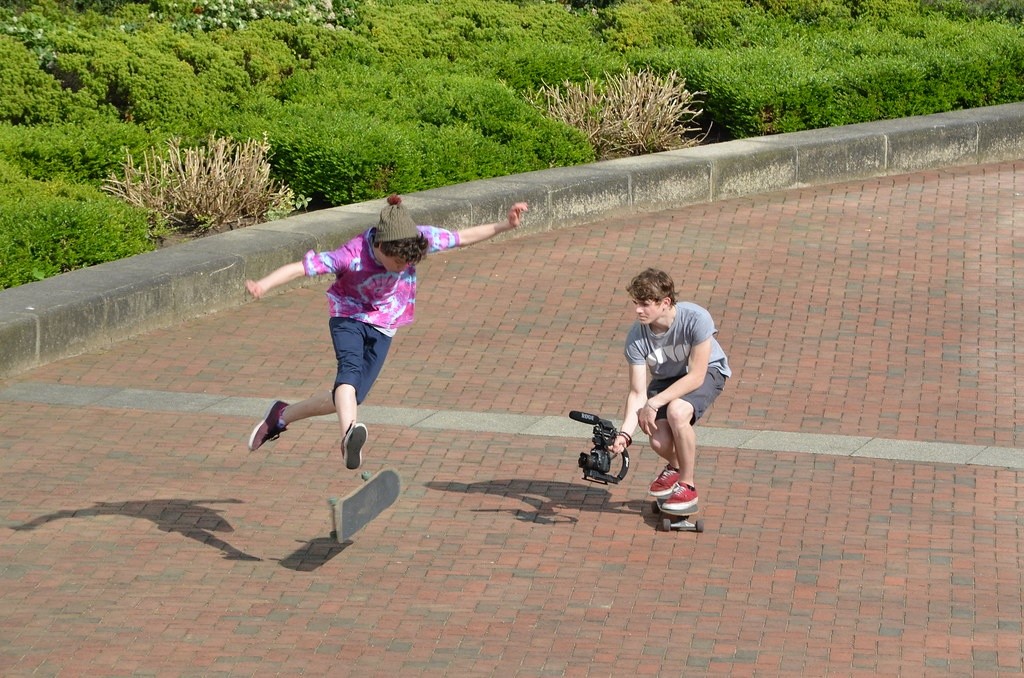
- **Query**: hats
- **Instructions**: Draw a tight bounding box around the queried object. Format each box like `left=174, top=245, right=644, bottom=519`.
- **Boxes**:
left=374, top=195, right=418, bottom=242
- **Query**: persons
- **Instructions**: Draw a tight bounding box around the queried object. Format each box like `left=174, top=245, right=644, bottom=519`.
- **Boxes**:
left=605, top=268, right=731, bottom=511
left=247, top=193, right=529, bottom=470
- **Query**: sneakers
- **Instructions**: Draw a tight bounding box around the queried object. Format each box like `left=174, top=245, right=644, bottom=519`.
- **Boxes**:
left=249, top=400, right=290, bottom=451
left=662, top=482, right=698, bottom=510
left=341, top=422, right=368, bottom=470
left=648, top=464, right=681, bottom=496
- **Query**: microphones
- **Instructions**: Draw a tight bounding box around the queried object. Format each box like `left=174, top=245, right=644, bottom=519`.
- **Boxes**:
left=568, top=411, right=601, bottom=425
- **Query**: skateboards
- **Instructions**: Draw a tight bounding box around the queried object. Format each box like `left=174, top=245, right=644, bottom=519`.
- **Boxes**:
left=650, top=492, right=704, bottom=533
left=327, top=466, right=403, bottom=544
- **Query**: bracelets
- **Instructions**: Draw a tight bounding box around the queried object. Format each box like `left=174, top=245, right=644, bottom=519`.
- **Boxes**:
left=646, top=399, right=659, bottom=412
left=618, top=431, right=632, bottom=447
